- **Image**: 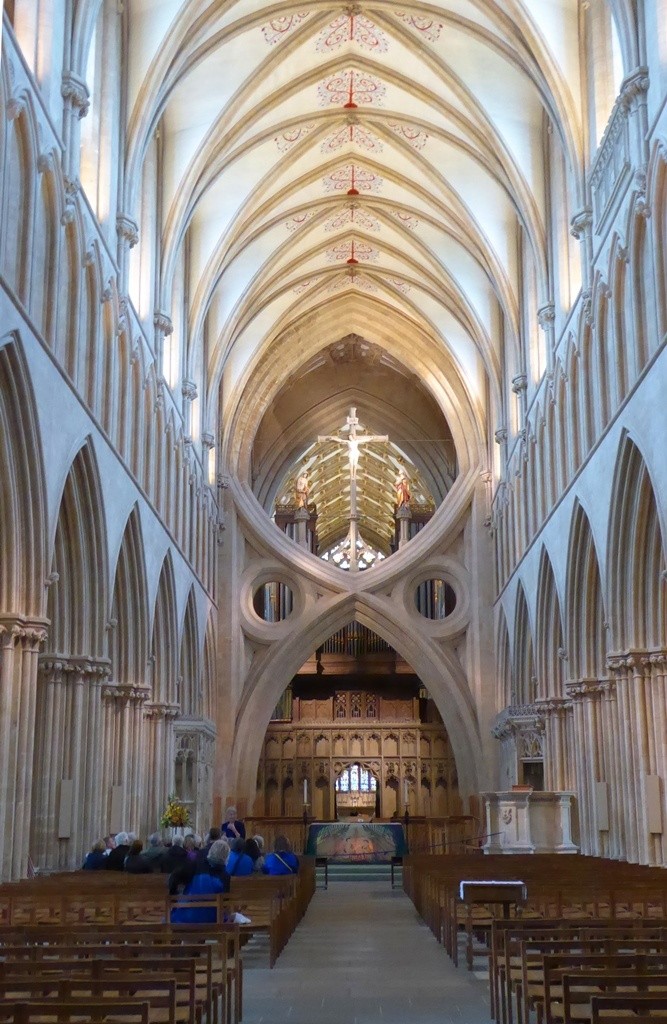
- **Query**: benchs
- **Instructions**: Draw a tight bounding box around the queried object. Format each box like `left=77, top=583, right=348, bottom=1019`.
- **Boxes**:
left=403, top=854, right=667, bottom=1024
left=0, top=855, right=317, bottom=1024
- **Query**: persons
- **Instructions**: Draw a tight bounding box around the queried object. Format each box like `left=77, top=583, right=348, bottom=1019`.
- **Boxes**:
left=106, top=832, right=131, bottom=870
left=124, top=840, right=153, bottom=873
left=162, top=840, right=231, bottom=944
left=228, top=835, right=264, bottom=876
left=128, top=832, right=136, bottom=846
left=330, top=434, right=373, bottom=480
left=261, top=834, right=300, bottom=878
left=156, top=828, right=221, bottom=874
left=141, top=832, right=166, bottom=862
left=221, top=805, right=245, bottom=839
left=395, top=469, right=410, bottom=509
left=295, top=471, right=307, bottom=512
left=83, top=834, right=117, bottom=869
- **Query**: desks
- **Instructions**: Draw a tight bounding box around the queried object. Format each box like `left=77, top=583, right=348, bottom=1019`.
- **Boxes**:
left=307, top=822, right=408, bottom=891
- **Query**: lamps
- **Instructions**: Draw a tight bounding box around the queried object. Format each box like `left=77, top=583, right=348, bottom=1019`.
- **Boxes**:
left=304, top=779, right=307, bottom=804
left=405, top=779, right=408, bottom=802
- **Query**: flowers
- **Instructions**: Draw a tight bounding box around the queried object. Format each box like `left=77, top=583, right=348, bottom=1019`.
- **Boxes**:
left=159, top=792, right=198, bottom=828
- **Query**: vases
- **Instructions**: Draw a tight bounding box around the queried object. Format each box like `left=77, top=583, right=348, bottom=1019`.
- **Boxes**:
left=167, top=825, right=192, bottom=848
